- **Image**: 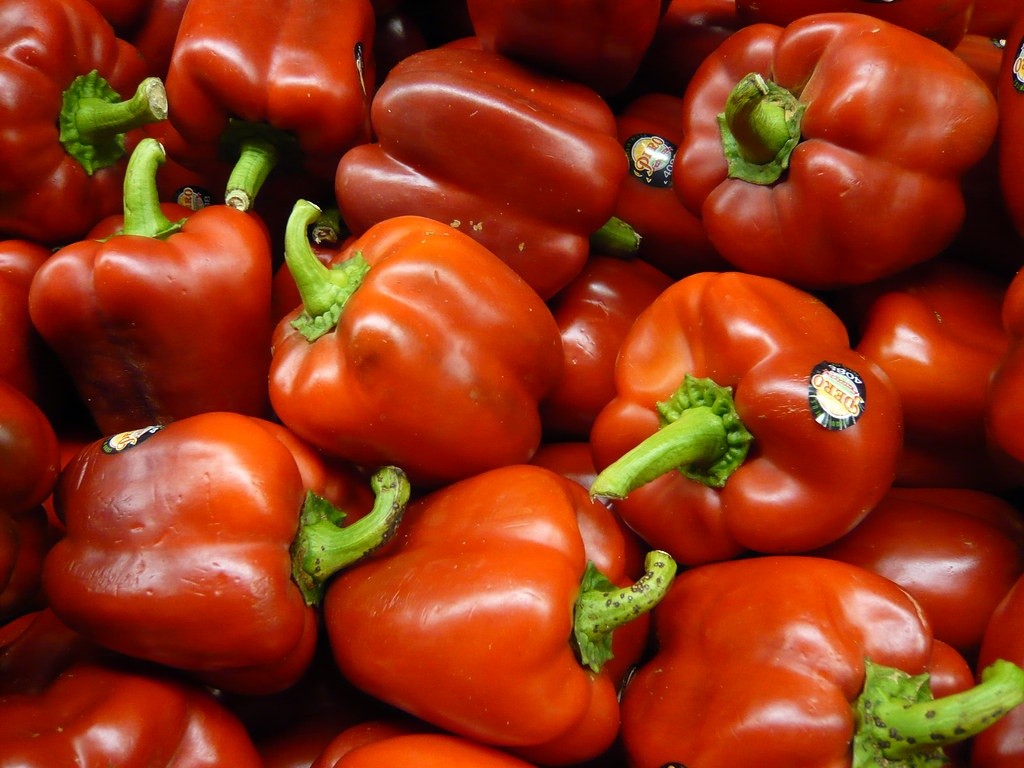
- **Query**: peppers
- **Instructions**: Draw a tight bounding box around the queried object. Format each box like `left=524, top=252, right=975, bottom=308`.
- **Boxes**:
left=0, top=0, right=1024, bottom=768
